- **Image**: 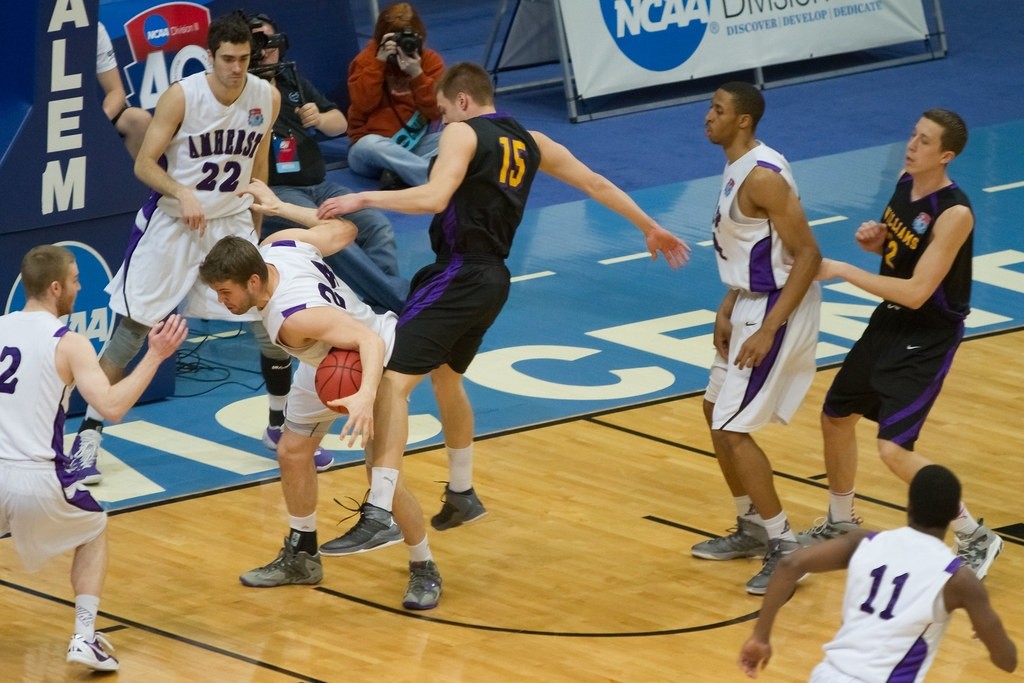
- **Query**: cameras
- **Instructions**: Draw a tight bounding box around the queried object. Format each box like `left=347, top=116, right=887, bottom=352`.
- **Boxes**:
left=389, top=26, right=423, bottom=57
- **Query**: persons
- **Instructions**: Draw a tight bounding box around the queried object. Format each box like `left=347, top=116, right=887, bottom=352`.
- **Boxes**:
left=0, top=245, right=189, bottom=671
left=347, top=2, right=447, bottom=191
left=65, top=14, right=334, bottom=486
left=198, top=178, right=443, bottom=610
left=234, top=10, right=411, bottom=313
left=691, top=81, right=821, bottom=595
left=738, top=464, right=1017, bottom=683
left=315, top=61, right=694, bottom=557
left=96, top=19, right=153, bottom=162
left=793, top=109, right=1005, bottom=583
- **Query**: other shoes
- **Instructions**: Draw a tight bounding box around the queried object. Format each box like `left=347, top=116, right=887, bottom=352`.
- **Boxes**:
left=379, top=170, right=404, bottom=190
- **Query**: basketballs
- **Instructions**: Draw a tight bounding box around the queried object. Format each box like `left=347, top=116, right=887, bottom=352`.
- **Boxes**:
left=315, top=348, right=362, bottom=415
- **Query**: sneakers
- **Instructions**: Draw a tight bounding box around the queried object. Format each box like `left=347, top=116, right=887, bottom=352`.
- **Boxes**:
left=692, top=516, right=769, bottom=560
left=955, top=517, right=1003, bottom=581
left=239, top=536, right=322, bottom=587
left=63, top=429, right=104, bottom=484
left=402, top=560, right=442, bottom=609
left=67, top=631, right=120, bottom=672
left=318, top=489, right=405, bottom=555
left=431, top=481, right=488, bottom=531
left=263, top=425, right=334, bottom=471
left=746, top=540, right=810, bottom=595
left=794, top=517, right=864, bottom=548
